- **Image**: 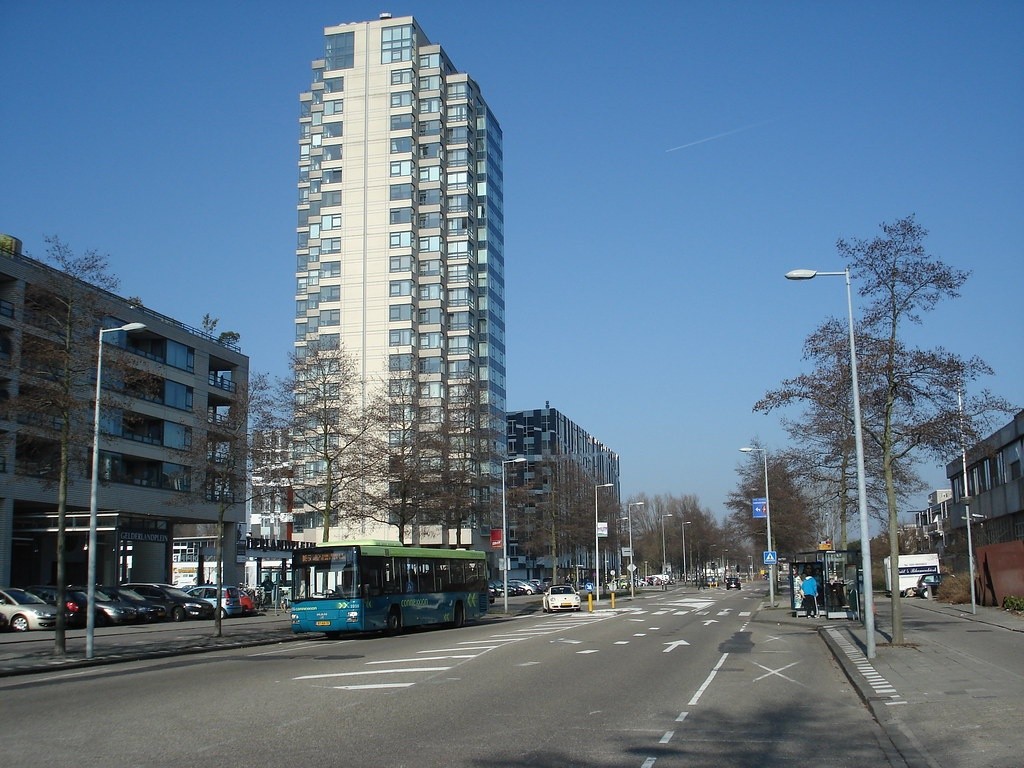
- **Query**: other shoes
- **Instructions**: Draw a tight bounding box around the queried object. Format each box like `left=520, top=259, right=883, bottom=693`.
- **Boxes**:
left=807, top=616, right=811, bottom=619
left=814, top=616, right=819, bottom=619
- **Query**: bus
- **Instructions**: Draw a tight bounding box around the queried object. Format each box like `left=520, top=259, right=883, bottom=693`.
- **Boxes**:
left=284, top=537, right=492, bottom=638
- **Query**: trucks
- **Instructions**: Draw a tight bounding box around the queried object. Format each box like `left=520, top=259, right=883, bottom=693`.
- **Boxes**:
left=883, top=552, right=940, bottom=598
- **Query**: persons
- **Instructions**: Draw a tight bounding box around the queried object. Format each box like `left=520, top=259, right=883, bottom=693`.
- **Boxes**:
left=261, top=576, right=273, bottom=607
left=792, top=565, right=822, bottom=609
left=802, top=570, right=818, bottom=618
left=698, top=575, right=705, bottom=590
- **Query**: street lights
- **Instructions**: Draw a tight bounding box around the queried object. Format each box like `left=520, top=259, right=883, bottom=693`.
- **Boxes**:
left=594, top=483, right=614, bottom=602
left=709, top=544, right=716, bottom=582
left=501, top=457, right=526, bottom=614
left=628, top=501, right=644, bottom=598
left=681, top=521, right=692, bottom=587
left=739, top=448, right=776, bottom=611
left=83, top=321, right=147, bottom=660
left=661, top=514, right=673, bottom=592
left=783, top=268, right=876, bottom=658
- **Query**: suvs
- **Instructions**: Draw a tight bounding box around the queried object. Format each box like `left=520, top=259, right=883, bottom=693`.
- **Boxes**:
left=917, top=573, right=950, bottom=600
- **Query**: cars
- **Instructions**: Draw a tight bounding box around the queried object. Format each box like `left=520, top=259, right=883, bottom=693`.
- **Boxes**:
left=66, top=585, right=138, bottom=627
left=184, top=584, right=256, bottom=619
left=120, top=582, right=215, bottom=622
left=25, top=584, right=86, bottom=628
left=487, top=575, right=594, bottom=606
left=541, top=585, right=582, bottom=613
left=706, top=576, right=719, bottom=586
left=607, top=573, right=672, bottom=589
left=0, top=587, right=69, bottom=632
left=85, top=584, right=167, bottom=624
left=725, top=577, right=742, bottom=590
